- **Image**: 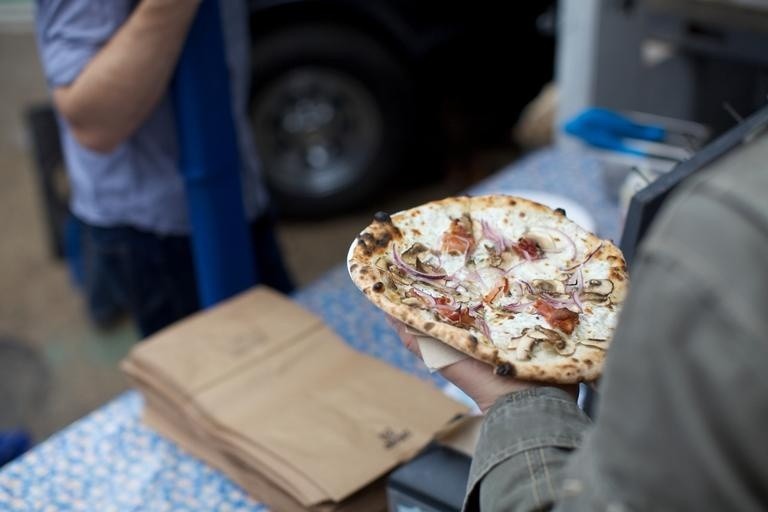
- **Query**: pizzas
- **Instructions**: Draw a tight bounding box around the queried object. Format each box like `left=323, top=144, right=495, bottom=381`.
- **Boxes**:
left=348, top=194, right=630, bottom=384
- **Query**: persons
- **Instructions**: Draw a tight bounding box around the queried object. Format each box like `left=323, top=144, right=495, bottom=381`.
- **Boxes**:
left=382, top=133, right=768, bottom=512
left=32, top=2, right=299, bottom=342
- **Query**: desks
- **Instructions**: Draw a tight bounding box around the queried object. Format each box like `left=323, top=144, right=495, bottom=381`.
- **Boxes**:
left=1, top=142, right=678, bottom=512
